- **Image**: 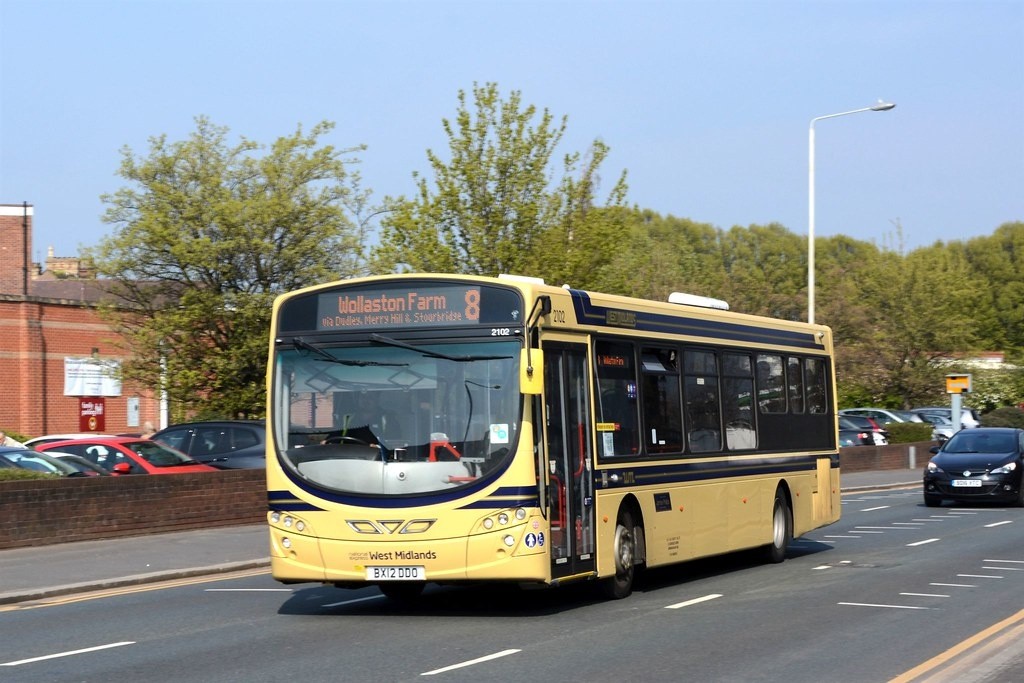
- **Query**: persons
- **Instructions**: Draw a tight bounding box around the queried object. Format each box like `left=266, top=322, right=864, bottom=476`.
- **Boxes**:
left=140, top=421, right=156, bottom=439
left=756, top=360, right=770, bottom=385
left=0, top=430, right=7, bottom=446
left=703, top=384, right=720, bottom=425
left=320, top=391, right=401, bottom=447
left=723, top=354, right=749, bottom=395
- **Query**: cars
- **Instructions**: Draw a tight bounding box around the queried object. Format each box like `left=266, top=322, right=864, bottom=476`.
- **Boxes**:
left=923, top=427, right=1024, bottom=507
left=0, top=432, right=216, bottom=475
left=838, top=404, right=984, bottom=448
left=125, top=419, right=265, bottom=470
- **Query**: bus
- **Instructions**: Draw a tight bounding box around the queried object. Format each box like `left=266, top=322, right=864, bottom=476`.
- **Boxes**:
left=264, top=271, right=840, bottom=594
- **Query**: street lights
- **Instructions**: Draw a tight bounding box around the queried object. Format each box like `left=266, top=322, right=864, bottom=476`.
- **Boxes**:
left=808, top=102, right=897, bottom=323
